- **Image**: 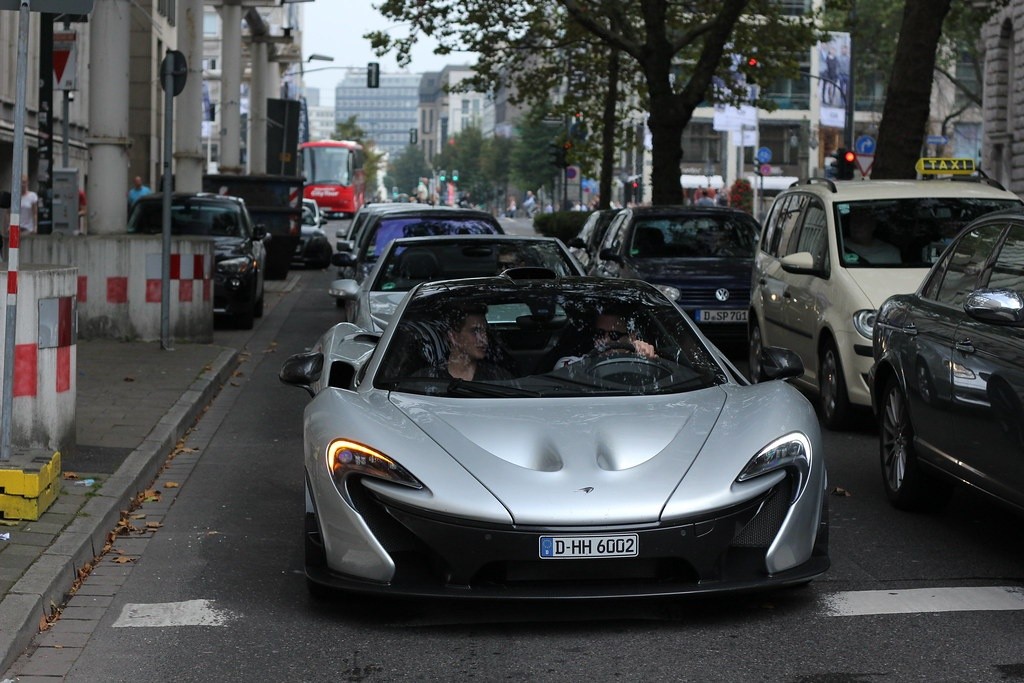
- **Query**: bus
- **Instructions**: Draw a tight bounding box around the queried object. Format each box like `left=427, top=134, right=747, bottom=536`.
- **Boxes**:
left=297, top=138, right=366, bottom=219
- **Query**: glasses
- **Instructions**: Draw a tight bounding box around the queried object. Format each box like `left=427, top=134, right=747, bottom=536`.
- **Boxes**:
left=591, top=328, right=636, bottom=341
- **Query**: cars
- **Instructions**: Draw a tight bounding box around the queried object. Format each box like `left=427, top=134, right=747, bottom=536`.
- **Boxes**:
left=565, top=206, right=621, bottom=277
left=594, top=204, right=762, bottom=359
left=869, top=205, right=1024, bottom=537
left=289, top=197, right=509, bottom=308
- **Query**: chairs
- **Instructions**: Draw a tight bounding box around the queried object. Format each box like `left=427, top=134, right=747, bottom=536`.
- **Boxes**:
left=400, top=248, right=441, bottom=280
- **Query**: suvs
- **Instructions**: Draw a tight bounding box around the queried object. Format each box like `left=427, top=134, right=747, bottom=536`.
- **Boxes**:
left=746, top=157, right=1024, bottom=432
left=126, top=190, right=274, bottom=331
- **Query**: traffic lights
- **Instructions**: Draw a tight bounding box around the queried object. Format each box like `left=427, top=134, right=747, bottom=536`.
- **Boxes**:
left=548, top=142, right=561, bottom=168
left=746, top=52, right=758, bottom=85
left=830, top=148, right=843, bottom=180
left=843, top=151, right=856, bottom=180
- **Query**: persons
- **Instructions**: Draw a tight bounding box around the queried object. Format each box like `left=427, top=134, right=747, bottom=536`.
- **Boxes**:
left=506, top=196, right=516, bottom=218
left=4, top=175, right=39, bottom=234
left=818, top=39, right=850, bottom=108
left=697, top=191, right=716, bottom=208
left=693, top=183, right=715, bottom=199
left=411, top=303, right=514, bottom=382
left=713, top=189, right=730, bottom=208
left=549, top=310, right=662, bottom=372
left=128, top=175, right=151, bottom=207
left=521, top=191, right=537, bottom=217
left=78, top=191, right=87, bottom=230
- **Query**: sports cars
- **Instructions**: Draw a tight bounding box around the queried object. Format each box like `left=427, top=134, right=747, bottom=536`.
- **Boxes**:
left=277, top=265, right=835, bottom=608
left=328, top=231, right=603, bottom=340
